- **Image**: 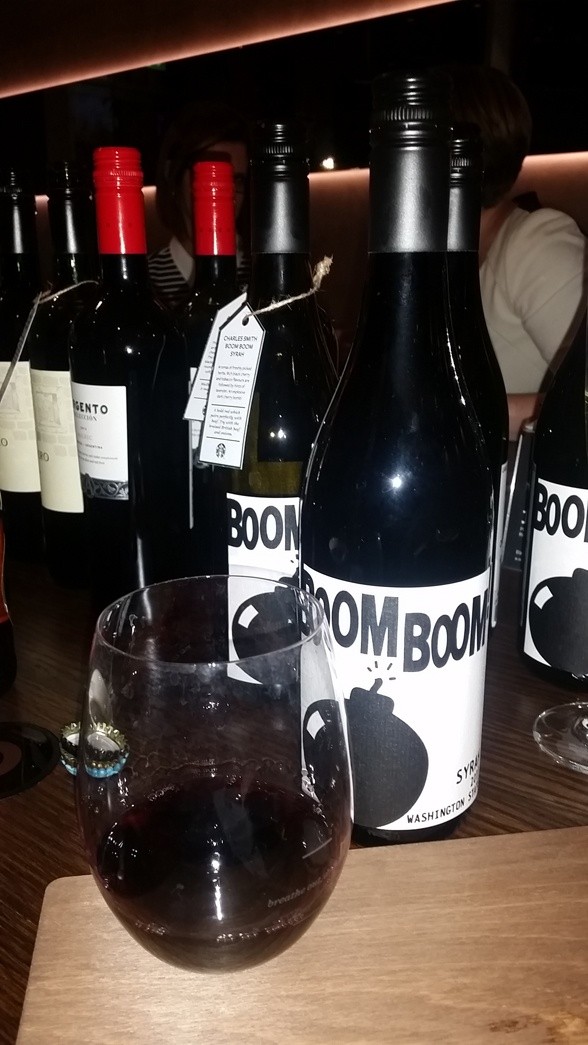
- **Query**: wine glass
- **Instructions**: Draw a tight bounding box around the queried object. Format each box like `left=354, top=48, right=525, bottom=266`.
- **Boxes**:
left=532, top=701, right=588, bottom=774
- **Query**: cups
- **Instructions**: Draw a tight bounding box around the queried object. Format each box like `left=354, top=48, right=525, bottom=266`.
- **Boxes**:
left=76, top=574, right=355, bottom=976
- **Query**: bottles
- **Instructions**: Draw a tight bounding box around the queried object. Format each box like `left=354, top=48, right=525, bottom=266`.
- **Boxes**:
left=520, top=284, right=588, bottom=693
left=0, top=122, right=341, bottom=707
left=297, top=73, right=509, bottom=847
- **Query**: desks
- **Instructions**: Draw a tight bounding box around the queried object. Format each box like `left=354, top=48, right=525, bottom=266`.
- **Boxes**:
left=0, top=604, right=588, bottom=1045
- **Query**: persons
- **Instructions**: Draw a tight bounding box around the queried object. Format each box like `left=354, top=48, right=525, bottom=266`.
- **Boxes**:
left=138, top=108, right=253, bottom=314
left=436, top=58, right=586, bottom=445
